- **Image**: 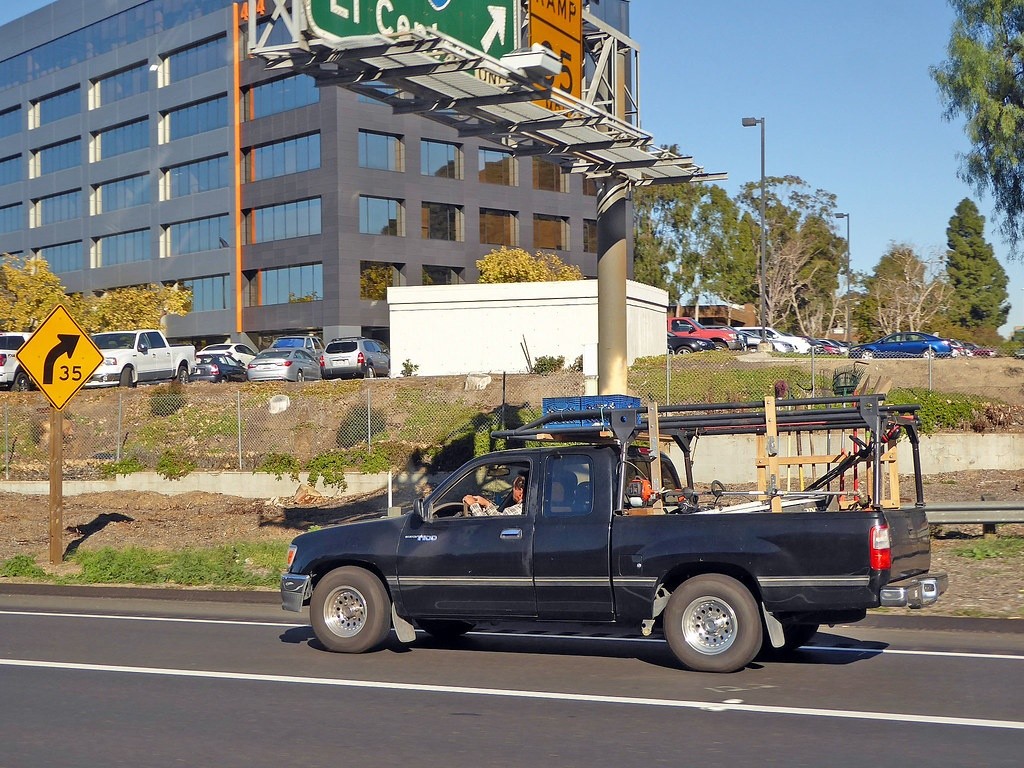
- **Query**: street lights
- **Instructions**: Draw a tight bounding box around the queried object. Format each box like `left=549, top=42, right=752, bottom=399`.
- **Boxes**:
left=833, top=212, right=852, bottom=338
left=741, top=116, right=774, bottom=354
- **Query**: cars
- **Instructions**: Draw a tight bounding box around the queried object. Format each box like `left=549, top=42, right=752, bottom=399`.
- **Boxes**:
left=848, top=331, right=996, bottom=360
left=665, top=316, right=852, bottom=355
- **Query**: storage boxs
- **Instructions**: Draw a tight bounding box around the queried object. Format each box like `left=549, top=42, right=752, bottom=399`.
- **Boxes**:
left=541, top=394, right=641, bottom=428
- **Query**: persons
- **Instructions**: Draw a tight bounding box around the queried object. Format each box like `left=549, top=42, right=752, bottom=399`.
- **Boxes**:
left=463, top=475, right=527, bottom=518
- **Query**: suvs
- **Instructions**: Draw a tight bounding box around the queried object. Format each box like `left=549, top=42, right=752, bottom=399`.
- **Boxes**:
left=200, top=332, right=391, bottom=380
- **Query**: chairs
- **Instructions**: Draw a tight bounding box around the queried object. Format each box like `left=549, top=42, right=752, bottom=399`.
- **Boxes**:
left=549, top=482, right=574, bottom=512
left=572, top=481, right=591, bottom=513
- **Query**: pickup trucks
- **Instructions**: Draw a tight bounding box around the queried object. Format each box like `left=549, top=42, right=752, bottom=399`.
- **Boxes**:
left=280, top=368, right=948, bottom=671
left=0, top=325, right=204, bottom=390
